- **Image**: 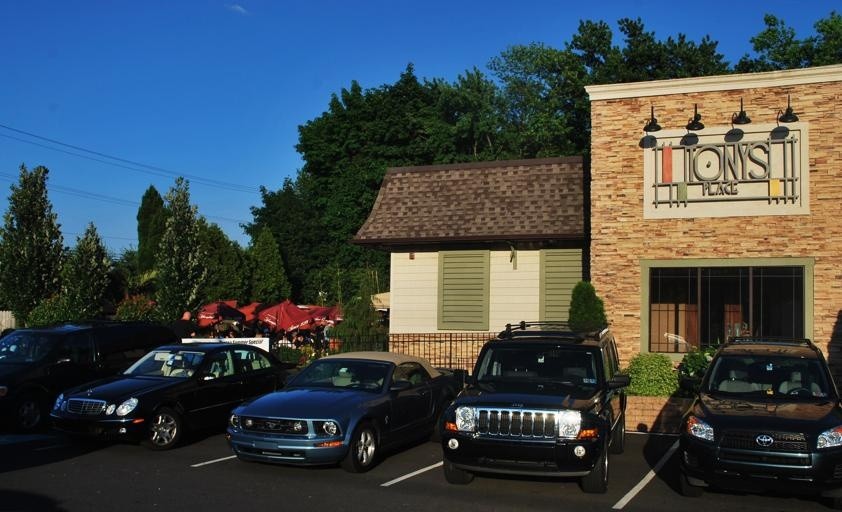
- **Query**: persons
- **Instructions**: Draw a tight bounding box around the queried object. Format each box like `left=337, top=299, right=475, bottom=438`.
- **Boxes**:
left=174, top=311, right=335, bottom=353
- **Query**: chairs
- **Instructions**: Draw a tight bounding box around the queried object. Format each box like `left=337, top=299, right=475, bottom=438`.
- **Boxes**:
left=778, top=368, right=825, bottom=395
left=718, top=364, right=762, bottom=393
left=161, top=353, right=263, bottom=379
left=332, top=368, right=354, bottom=389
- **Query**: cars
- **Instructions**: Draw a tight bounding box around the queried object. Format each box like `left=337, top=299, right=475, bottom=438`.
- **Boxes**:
left=46, top=340, right=301, bottom=454
left=222, top=349, right=469, bottom=475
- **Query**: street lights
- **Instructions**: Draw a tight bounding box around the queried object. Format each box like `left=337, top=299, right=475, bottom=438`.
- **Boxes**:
left=318, top=290, right=329, bottom=307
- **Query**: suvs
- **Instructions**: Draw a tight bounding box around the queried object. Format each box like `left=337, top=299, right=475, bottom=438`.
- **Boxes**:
left=677, top=334, right=842, bottom=500
left=440, top=318, right=632, bottom=494
left=0, top=317, right=180, bottom=434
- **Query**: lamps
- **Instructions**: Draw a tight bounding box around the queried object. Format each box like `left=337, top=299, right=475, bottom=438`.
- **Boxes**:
left=642, top=105, right=663, bottom=134
left=734, top=97, right=753, bottom=124
left=778, top=91, right=801, bottom=124
left=686, top=102, right=704, bottom=130
